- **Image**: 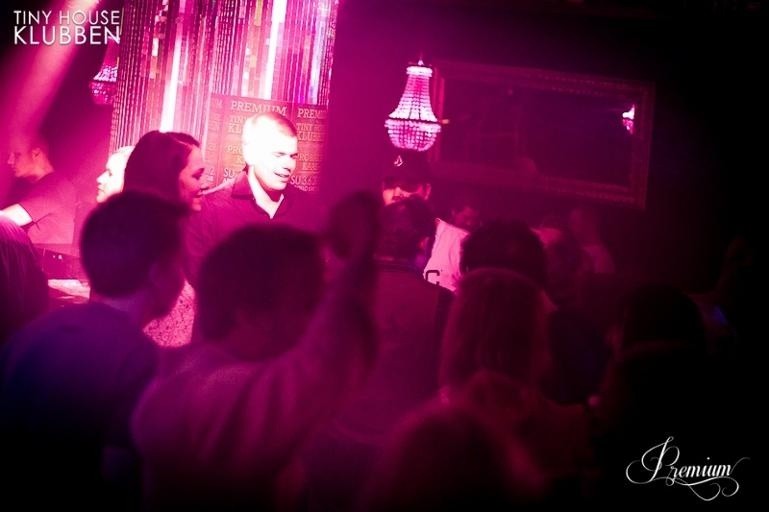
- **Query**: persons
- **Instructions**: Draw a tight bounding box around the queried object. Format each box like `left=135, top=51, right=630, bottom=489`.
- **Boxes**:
left=93, top=143, right=136, bottom=203
left=0, top=188, right=768, bottom=511
left=375, top=145, right=474, bottom=295
left=0, top=125, right=78, bottom=249
left=176, top=110, right=334, bottom=274
left=120, top=128, right=210, bottom=349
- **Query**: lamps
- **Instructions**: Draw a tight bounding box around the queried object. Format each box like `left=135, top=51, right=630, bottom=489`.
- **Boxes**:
left=384, top=69, right=449, bottom=152
